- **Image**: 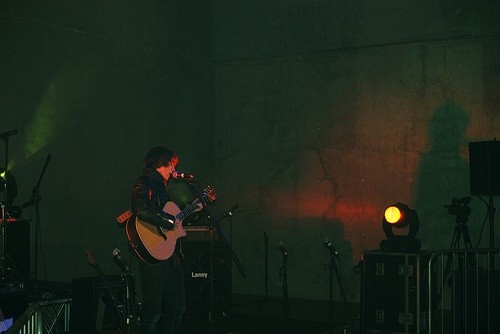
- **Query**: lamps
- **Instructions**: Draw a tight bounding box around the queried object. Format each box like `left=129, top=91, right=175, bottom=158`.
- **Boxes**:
left=380, top=202, right=422, bottom=253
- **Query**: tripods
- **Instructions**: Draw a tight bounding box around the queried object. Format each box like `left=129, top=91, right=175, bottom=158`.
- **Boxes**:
left=434, top=224, right=477, bottom=328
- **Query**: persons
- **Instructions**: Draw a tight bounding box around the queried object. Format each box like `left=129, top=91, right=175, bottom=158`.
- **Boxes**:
left=131, top=146, right=207, bottom=334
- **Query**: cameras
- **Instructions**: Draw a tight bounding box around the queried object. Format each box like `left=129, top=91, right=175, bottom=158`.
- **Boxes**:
left=444, top=197, right=472, bottom=224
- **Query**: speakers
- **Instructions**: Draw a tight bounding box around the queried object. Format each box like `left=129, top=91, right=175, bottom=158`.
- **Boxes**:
left=360, top=251, right=434, bottom=334
left=0, top=274, right=136, bottom=334
left=177, top=238, right=232, bottom=319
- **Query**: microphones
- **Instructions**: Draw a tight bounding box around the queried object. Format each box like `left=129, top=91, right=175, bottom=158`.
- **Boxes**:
left=279, top=241, right=287, bottom=255
left=22, top=196, right=41, bottom=208
left=324, top=240, right=338, bottom=255
left=0, top=129, right=17, bottom=139
left=223, top=202, right=238, bottom=216
left=173, top=172, right=193, bottom=178
left=113, top=249, right=129, bottom=271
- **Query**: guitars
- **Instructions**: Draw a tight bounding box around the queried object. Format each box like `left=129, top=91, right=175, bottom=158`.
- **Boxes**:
left=125, top=184, right=218, bottom=265
left=86, top=249, right=137, bottom=334
